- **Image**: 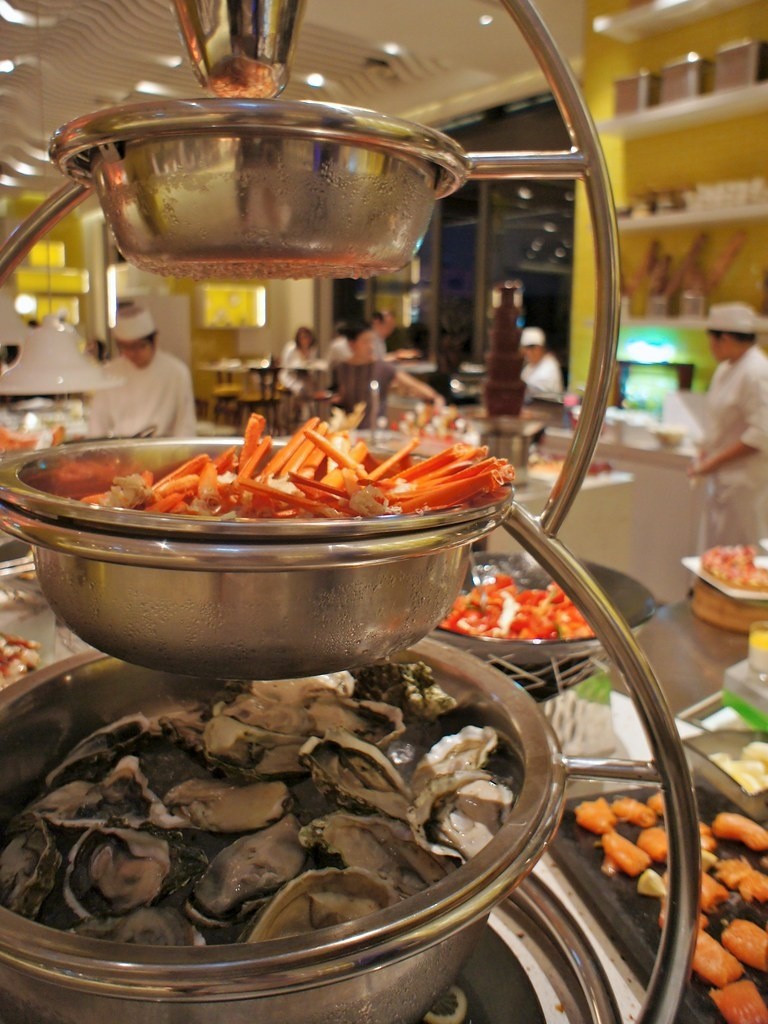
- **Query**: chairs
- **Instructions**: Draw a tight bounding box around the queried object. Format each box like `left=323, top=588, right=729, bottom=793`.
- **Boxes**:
left=209, top=357, right=398, bottom=438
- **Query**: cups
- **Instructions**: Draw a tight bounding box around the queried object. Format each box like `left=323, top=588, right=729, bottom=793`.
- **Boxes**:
left=749, top=620, right=768, bottom=685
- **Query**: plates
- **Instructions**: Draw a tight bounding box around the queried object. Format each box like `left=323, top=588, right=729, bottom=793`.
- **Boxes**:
left=681, top=556, right=768, bottom=600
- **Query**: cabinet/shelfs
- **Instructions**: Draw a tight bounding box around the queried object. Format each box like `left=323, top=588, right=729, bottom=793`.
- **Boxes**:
left=591, top=0, right=768, bottom=234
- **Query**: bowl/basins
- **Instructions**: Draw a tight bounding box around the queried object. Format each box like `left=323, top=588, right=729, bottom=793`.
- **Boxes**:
left=425, top=551, right=654, bottom=666
left=0, top=436, right=515, bottom=679
left=47, top=100, right=473, bottom=278
left=0, top=638, right=568, bottom=1024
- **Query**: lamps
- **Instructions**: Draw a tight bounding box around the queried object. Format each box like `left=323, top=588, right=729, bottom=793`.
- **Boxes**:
left=200, top=282, right=266, bottom=331
left=16, top=238, right=88, bottom=326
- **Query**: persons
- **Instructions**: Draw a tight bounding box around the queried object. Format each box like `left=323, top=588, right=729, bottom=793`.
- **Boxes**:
left=519, top=327, right=563, bottom=404
left=328, top=310, right=446, bottom=429
left=277, top=327, right=319, bottom=396
left=87, top=307, right=197, bottom=436
left=685, top=303, right=768, bottom=596
left=5, top=308, right=80, bottom=402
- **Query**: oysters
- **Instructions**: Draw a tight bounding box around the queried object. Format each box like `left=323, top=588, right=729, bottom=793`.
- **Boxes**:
left=0, top=652, right=516, bottom=946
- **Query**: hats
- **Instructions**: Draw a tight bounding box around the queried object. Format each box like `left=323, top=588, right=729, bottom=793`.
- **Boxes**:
left=520, top=326, right=545, bottom=347
left=110, top=304, right=157, bottom=342
left=705, top=301, right=760, bottom=333
